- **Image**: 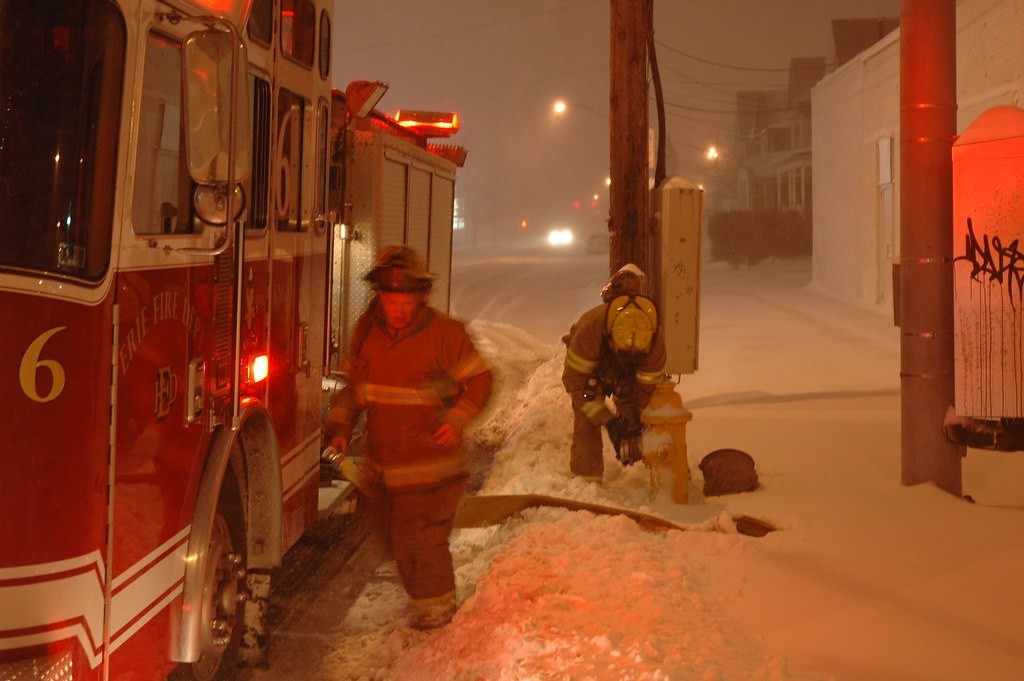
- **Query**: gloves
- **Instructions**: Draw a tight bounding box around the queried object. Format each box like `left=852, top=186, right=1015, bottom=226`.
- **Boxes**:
left=607, top=418, right=626, bottom=460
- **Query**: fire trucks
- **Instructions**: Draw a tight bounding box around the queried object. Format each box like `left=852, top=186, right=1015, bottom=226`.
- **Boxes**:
left=0, top=1, right=467, bottom=681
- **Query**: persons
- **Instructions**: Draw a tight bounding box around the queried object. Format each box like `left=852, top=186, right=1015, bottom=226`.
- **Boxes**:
left=564, top=295, right=667, bottom=489
left=325, top=246, right=496, bottom=629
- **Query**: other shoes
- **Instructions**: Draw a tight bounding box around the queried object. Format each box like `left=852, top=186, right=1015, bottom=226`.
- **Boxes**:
left=413, top=609, right=453, bottom=628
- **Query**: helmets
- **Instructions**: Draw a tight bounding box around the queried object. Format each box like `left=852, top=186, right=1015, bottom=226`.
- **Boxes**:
left=361, top=247, right=439, bottom=292
left=612, top=309, right=653, bottom=355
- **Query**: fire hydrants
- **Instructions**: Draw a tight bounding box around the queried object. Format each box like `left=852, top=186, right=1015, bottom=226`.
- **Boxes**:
left=620, top=374, right=690, bottom=508
